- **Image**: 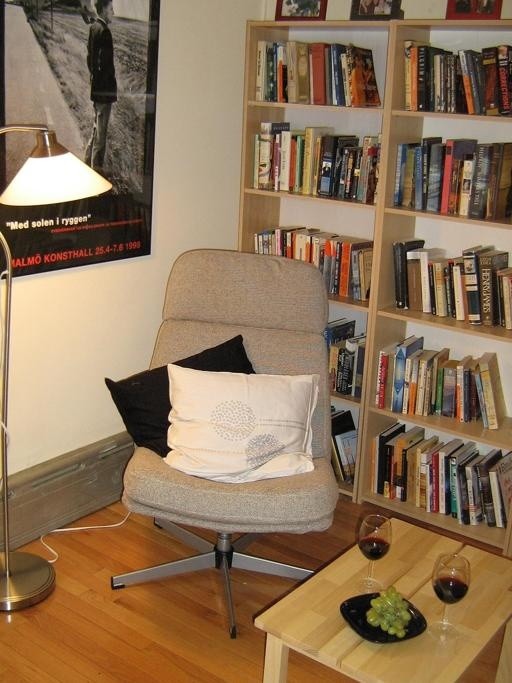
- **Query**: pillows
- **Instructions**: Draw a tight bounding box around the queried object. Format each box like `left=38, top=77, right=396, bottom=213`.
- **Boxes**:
left=88, top=331, right=324, bottom=487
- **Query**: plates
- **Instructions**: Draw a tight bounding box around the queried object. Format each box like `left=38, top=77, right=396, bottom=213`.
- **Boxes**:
left=339, top=593, right=428, bottom=644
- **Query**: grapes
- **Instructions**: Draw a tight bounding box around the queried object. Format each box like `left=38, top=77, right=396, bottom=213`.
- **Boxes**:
left=366, top=586, right=411, bottom=638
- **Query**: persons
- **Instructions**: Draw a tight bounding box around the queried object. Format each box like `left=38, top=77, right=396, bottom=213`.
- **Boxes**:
left=83, top=0, right=119, bottom=177
left=359, top=0, right=379, bottom=16
left=373, top=0, right=392, bottom=16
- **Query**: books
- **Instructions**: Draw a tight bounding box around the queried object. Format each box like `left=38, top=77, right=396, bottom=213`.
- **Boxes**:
left=376, top=334, right=507, bottom=429
left=256, top=41, right=381, bottom=107
left=404, top=41, right=512, bottom=115
left=254, top=123, right=383, bottom=203
left=330, top=406, right=358, bottom=485
left=371, top=422, right=512, bottom=527
left=394, top=137, right=512, bottom=218
left=393, top=238, right=512, bottom=329
left=326, top=318, right=366, bottom=398
left=253, top=226, right=373, bottom=301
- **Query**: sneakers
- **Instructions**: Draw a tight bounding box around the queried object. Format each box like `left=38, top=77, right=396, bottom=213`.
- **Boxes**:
left=88, top=165, right=114, bottom=178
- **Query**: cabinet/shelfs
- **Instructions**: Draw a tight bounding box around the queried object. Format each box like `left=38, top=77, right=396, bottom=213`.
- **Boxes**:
left=354, top=19, right=512, bottom=546
left=235, top=18, right=390, bottom=502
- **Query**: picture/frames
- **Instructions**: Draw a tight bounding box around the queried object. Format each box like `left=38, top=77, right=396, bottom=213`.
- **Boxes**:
left=1, top=0, right=163, bottom=277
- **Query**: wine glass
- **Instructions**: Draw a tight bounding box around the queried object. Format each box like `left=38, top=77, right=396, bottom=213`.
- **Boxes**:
left=357, top=513, right=393, bottom=594
left=428, top=552, right=472, bottom=642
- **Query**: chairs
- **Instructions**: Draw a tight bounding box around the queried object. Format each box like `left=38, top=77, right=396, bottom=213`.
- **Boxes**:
left=113, top=243, right=341, bottom=637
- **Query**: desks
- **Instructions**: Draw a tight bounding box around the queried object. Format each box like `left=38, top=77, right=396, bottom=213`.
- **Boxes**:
left=253, top=517, right=512, bottom=683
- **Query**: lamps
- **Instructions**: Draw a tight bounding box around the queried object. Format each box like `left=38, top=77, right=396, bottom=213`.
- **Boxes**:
left=0, top=123, right=111, bottom=618
